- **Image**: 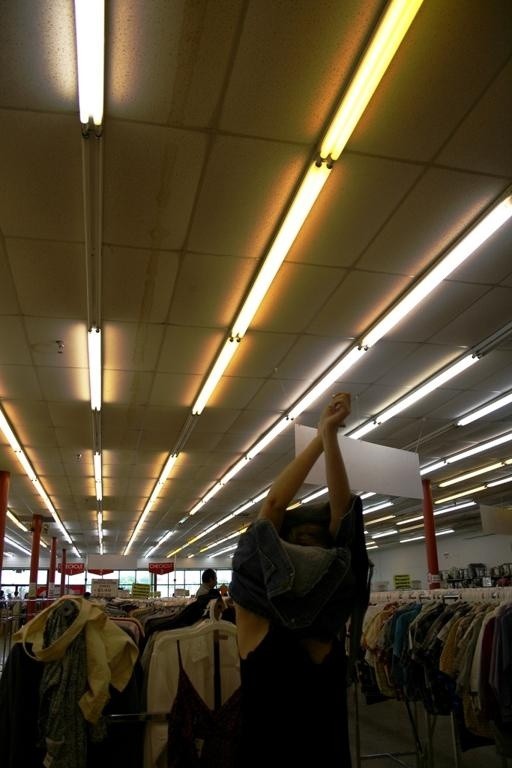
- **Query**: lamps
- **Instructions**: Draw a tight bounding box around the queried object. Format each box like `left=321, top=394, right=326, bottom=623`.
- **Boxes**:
left=0, top=0, right=512, bottom=560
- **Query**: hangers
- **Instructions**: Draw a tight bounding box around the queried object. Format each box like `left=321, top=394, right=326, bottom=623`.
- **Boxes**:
left=370, top=585, right=512, bottom=613
left=145, top=598, right=237, bottom=652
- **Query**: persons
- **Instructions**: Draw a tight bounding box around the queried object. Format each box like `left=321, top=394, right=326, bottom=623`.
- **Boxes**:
left=228, top=385, right=373, bottom=768
left=196, top=569, right=227, bottom=596
left=85, top=592, right=90, bottom=599
left=0, top=591, right=28, bottom=614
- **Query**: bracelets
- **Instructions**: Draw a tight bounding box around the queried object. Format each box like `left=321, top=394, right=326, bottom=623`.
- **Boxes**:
left=218, top=590, right=222, bottom=594
